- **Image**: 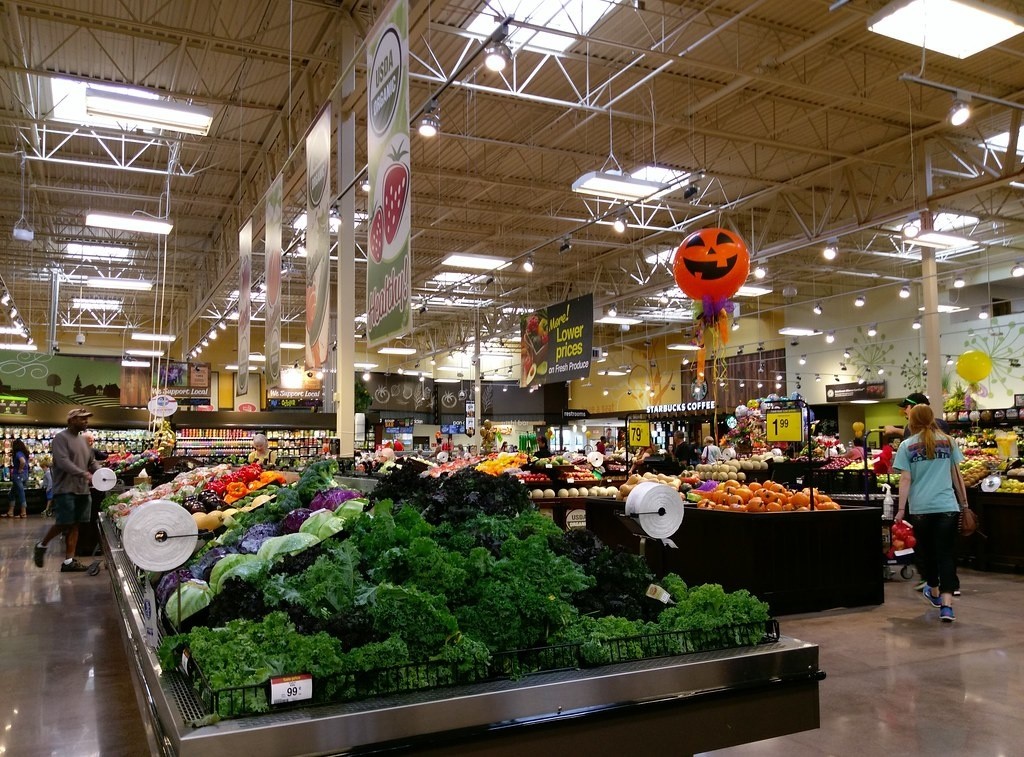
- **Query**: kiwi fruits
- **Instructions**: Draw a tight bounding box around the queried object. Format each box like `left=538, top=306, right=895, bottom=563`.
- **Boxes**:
left=961, top=467, right=989, bottom=487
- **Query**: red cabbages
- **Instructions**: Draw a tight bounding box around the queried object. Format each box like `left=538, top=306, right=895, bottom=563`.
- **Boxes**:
left=157, top=487, right=361, bottom=603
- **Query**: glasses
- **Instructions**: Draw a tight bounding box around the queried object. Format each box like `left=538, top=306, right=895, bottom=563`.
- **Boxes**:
left=903, top=397, right=918, bottom=405
left=70, top=409, right=86, bottom=415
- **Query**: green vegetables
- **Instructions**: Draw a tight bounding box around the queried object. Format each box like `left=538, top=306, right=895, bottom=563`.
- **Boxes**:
left=156, top=634, right=190, bottom=671
left=524, top=510, right=770, bottom=668
left=186, top=457, right=537, bottom=727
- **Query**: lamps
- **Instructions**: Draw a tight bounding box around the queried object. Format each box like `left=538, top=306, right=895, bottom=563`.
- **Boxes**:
left=0, top=26, right=1024, bottom=401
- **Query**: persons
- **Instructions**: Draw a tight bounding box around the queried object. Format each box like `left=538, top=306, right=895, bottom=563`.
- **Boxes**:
left=430, top=438, right=442, bottom=459
left=500, top=441, right=509, bottom=453
left=247, top=433, right=276, bottom=465
left=534, top=436, right=551, bottom=458
left=394, top=436, right=404, bottom=451
left=673, top=431, right=691, bottom=466
left=893, top=403, right=969, bottom=620
left=700, top=436, right=721, bottom=464
left=597, top=436, right=606, bottom=455
left=34, top=409, right=108, bottom=571
left=0, top=439, right=30, bottom=518
left=629, top=442, right=655, bottom=475
left=882, top=393, right=961, bottom=595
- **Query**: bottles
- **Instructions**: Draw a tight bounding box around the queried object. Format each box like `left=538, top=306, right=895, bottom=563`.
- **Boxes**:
left=177, top=440, right=184, bottom=446
left=200, top=439, right=228, bottom=446
left=99, top=431, right=113, bottom=453
left=242, top=440, right=254, bottom=447
left=175, top=449, right=185, bottom=456
left=126, top=431, right=136, bottom=452
left=233, top=429, right=255, bottom=437
left=187, top=449, right=200, bottom=456
left=194, top=457, right=209, bottom=463
left=176, top=429, right=202, bottom=437
left=203, top=429, right=232, bottom=437
left=113, top=432, right=125, bottom=452
left=87, top=430, right=98, bottom=448
left=811, top=423, right=816, bottom=434
left=804, top=424, right=808, bottom=440
left=956, top=437, right=967, bottom=449
left=240, top=448, right=255, bottom=456
left=228, top=449, right=239, bottom=456
left=137, top=431, right=153, bottom=453
left=211, top=449, right=228, bottom=456
left=200, top=449, right=211, bottom=456
left=185, top=440, right=199, bottom=446
left=229, top=439, right=242, bottom=447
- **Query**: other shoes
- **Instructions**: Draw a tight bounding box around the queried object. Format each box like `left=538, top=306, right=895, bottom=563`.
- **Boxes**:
left=1, top=512, right=13, bottom=517
left=61, top=558, right=89, bottom=572
left=15, top=513, right=27, bottom=518
left=954, top=589, right=961, bottom=596
left=35, top=541, right=47, bottom=567
left=41, top=508, right=54, bottom=515
left=914, top=576, right=941, bottom=590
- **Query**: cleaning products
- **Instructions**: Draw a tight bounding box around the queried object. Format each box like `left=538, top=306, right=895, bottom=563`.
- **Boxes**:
left=882, top=482, right=893, bottom=517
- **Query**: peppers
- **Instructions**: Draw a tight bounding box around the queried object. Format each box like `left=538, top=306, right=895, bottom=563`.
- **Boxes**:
left=526, top=316, right=549, bottom=353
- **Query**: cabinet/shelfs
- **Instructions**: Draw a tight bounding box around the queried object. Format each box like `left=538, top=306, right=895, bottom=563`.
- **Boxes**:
left=0, top=426, right=335, bottom=515
left=517, top=406, right=1024, bottom=619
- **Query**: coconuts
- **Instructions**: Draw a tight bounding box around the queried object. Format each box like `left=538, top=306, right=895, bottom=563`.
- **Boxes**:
left=535, top=456, right=564, bottom=466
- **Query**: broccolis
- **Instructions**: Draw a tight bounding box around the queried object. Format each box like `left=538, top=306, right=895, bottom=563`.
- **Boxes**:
left=297, top=458, right=340, bottom=498
left=263, top=487, right=303, bottom=519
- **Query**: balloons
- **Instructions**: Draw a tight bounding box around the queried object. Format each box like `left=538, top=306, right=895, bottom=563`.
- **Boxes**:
left=545, top=428, right=555, bottom=439
left=955, top=351, right=992, bottom=383
left=736, top=392, right=814, bottom=428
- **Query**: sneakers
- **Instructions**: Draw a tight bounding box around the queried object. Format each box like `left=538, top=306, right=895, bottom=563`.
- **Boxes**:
left=939, top=608, right=956, bottom=620
left=923, top=585, right=942, bottom=607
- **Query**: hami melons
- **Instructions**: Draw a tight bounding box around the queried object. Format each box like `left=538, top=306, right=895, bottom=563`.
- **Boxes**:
left=690, top=454, right=770, bottom=480
left=531, top=485, right=618, bottom=499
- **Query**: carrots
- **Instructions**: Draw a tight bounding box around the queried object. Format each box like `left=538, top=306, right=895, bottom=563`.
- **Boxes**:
left=107, top=462, right=232, bottom=521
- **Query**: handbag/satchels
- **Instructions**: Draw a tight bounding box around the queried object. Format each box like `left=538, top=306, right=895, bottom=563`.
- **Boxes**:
left=701, top=446, right=708, bottom=464
left=957, top=508, right=978, bottom=536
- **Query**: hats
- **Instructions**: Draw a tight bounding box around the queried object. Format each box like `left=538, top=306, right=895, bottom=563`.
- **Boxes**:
left=897, top=393, right=930, bottom=408
left=67, top=409, right=93, bottom=420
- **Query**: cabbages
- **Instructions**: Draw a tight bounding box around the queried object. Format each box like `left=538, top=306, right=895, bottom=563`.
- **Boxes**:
left=166, top=498, right=372, bottom=626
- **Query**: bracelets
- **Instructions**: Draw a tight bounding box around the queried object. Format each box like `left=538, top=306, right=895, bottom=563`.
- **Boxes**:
left=899, top=509, right=905, bottom=510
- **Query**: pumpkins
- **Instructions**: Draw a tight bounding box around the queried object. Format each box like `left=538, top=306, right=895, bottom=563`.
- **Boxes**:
left=617, top=472, right=687, bottom=504
left=696, top=478, right=841, bottom=512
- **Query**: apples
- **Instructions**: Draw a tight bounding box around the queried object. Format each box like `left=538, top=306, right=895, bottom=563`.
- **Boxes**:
left=793, top=457, right=901, bottom=488
left=959, top=459, right=983, bottom=475
left=995, top=479, right=1024, bottom=493
left=890, top=524, right=917, bottom=550
left=515, top=472, right=599, bottom=482
